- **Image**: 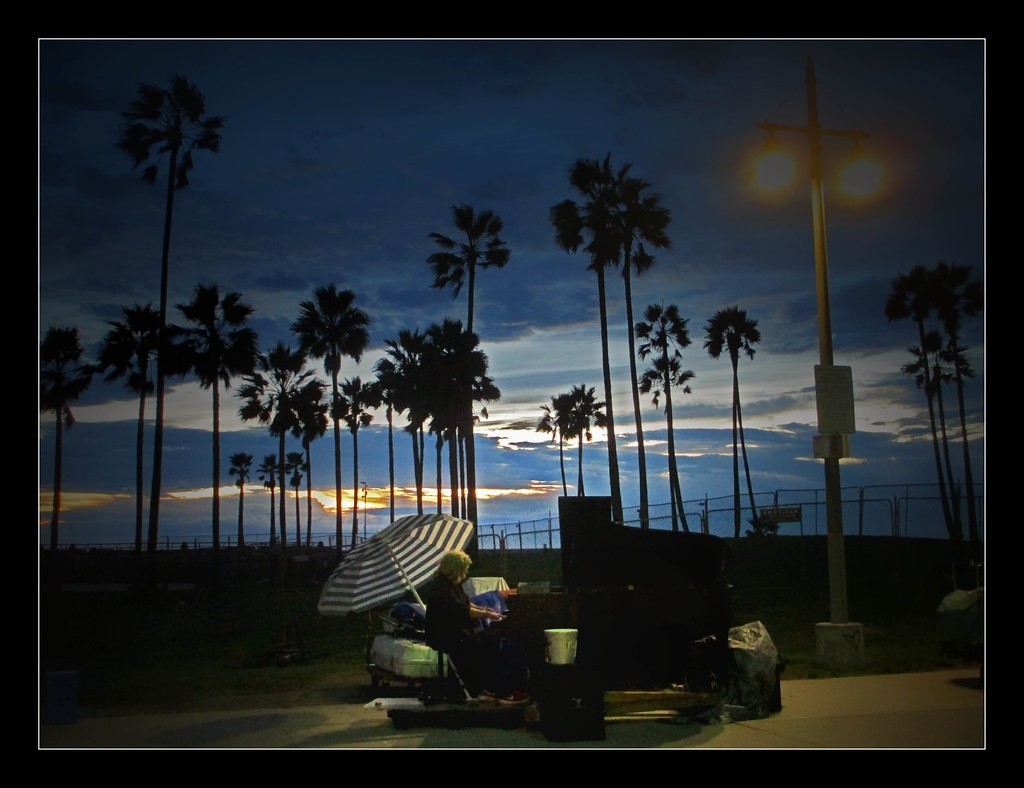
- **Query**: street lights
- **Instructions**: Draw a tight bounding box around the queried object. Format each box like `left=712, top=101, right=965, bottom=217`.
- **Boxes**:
left=755, top=123, right=887, bottom=675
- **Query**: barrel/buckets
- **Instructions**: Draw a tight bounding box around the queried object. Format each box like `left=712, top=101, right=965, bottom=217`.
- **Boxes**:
left=544, top=629, right=579, bottom=665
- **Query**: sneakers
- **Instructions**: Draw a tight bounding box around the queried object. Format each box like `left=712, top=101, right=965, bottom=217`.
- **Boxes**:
left=477, top=689, right=503, bottom=703
left=498, top=692, right=530, bottom=705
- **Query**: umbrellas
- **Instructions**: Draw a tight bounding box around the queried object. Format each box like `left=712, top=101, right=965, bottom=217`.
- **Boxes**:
left=318, top=513, right=475, bottom=697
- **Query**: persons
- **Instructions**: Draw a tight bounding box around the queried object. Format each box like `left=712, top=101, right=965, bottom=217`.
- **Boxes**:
left=425, top=549, right=531, bottom=704
